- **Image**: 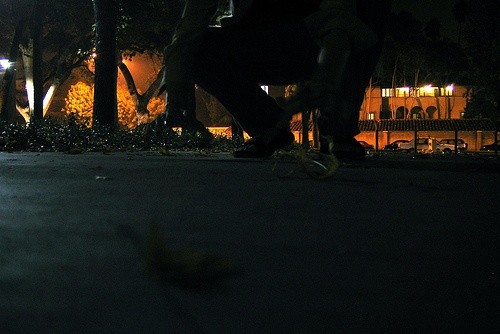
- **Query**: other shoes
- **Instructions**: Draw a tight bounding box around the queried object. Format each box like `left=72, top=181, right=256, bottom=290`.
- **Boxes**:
left=233, top=132, right=293, bottom=158
left=318, top=116, right=366, bottom=164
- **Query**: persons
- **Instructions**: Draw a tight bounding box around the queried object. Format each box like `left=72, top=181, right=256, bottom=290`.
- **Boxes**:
left=156, top=0, right=393, bottom=166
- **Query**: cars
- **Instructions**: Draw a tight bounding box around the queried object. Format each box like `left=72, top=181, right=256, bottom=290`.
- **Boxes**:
left=479, top=140, right=500, bottom=151
left=382, top=137, right=468, bottom=155
left=358, top=141, right=374, bottom=154
